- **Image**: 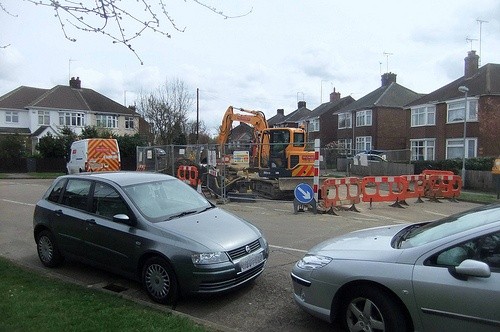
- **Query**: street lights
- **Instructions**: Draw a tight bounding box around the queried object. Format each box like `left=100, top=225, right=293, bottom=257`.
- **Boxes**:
left=457, top=87, right=469, bottom=188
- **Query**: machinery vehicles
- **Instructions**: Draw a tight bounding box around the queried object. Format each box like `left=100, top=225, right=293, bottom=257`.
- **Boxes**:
left=217, top=106, right=315, bottom=199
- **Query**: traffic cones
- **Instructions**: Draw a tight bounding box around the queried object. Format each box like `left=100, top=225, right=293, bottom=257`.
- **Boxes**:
left=196, top=179, right=202, bottom=196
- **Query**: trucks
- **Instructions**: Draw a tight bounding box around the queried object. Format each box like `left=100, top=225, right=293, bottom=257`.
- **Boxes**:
left=65, top=139, right=121, bottom=175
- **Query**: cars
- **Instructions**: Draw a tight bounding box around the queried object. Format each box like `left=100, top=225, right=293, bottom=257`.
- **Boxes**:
left=153, top=148, right=166, bottom=159
left=290, top=200, right=500, bottom=331
left=33, top=172, right=269, bottom=306
left=347, top=150, right=388, bottom=165
